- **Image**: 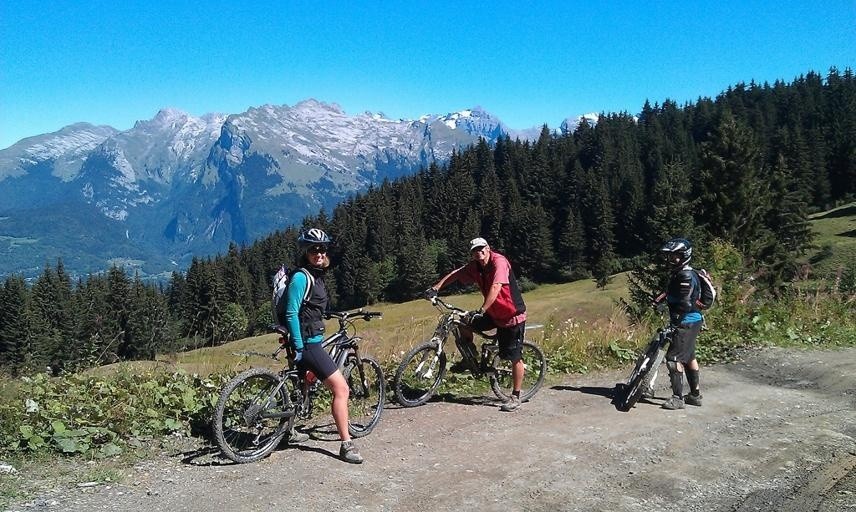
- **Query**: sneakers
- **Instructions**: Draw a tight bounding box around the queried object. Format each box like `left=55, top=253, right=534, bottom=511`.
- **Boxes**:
left=683, top=392, right=702, bottom=406
left=449, top=355, right=475, bottom=375
left=337, top=440, right=365, bottom=463
left=279, top=422, right=310, bottom=445
left=499, top=395, right=523, bottom=413
left=662, top=394, right=684, bottom=408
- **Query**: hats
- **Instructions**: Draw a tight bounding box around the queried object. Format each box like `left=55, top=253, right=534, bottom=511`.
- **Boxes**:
left=466, top=236, right=489, bottom=253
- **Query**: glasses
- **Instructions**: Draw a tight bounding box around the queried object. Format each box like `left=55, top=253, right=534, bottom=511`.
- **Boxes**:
left=307, top=246, right=327, bottom=256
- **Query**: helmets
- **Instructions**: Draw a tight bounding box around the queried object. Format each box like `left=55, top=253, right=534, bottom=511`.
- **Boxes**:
left=296, top=226, right=332, bottom=246
left=662, top=239, right=693, bottom=270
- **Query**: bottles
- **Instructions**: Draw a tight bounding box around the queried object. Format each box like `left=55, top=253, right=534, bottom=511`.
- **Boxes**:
left=469, top=341, right=479, bottom=357
left=307, top=372, right=317, bottom=383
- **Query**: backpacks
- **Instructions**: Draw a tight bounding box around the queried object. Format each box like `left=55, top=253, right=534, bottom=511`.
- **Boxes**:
left=269, top=264, right=315, bottom=335
left=689, top=267, right=717, bottom=312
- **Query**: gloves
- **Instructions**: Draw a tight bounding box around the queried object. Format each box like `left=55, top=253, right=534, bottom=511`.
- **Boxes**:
left=289, top=350, right=304, bottom=363
left=461, top=307, right=487, bottom=325
left=425, top=287, right=440, bottom=303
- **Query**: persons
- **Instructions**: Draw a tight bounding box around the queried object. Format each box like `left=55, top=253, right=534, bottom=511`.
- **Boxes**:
left=650, top=237, right=705, bottom=409
left=285, top=227, right=365, bottom=465
left=424, top=237, right=526, bottom=412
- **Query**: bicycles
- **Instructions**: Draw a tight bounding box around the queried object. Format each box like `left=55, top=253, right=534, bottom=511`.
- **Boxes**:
left=616, top=295, right=677, bottom=412
left=210, top=308, right=386, bottom=463
left=394, top=287, right=547, bottom=414
